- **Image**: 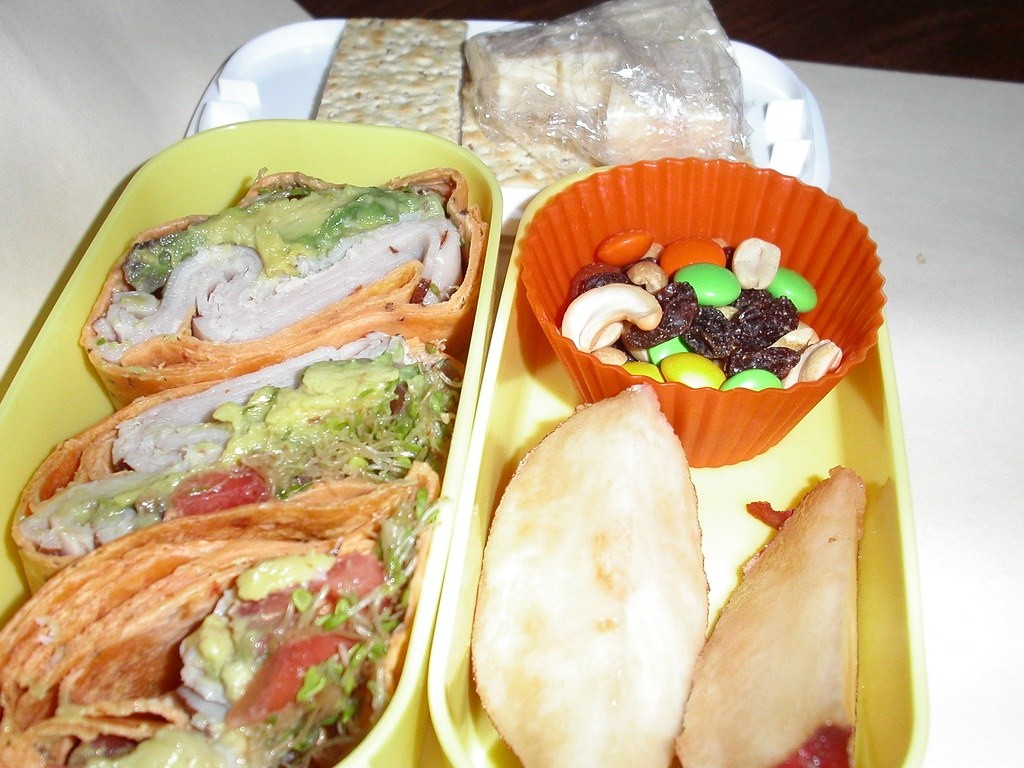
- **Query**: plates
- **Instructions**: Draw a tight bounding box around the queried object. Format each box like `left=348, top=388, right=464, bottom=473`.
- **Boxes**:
left=182, top=19, right=832, bottom=235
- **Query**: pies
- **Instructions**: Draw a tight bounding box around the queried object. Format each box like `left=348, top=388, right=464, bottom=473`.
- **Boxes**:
left=1, top=171, right=488, bottom=768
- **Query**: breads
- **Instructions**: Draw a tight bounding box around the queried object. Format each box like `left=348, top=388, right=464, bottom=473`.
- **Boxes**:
left=314, top=1, right=737, bottom=240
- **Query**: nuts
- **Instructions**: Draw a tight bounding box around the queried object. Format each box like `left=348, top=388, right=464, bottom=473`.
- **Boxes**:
left=554, top=237, right=844, bottom=390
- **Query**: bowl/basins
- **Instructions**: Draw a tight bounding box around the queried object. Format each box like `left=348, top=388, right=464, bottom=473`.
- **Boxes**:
left=429, top=166, right=928, bottom=767
left=1, top=119, right=503, bottom=767
left=516, top=157, right=887, bottom=466
